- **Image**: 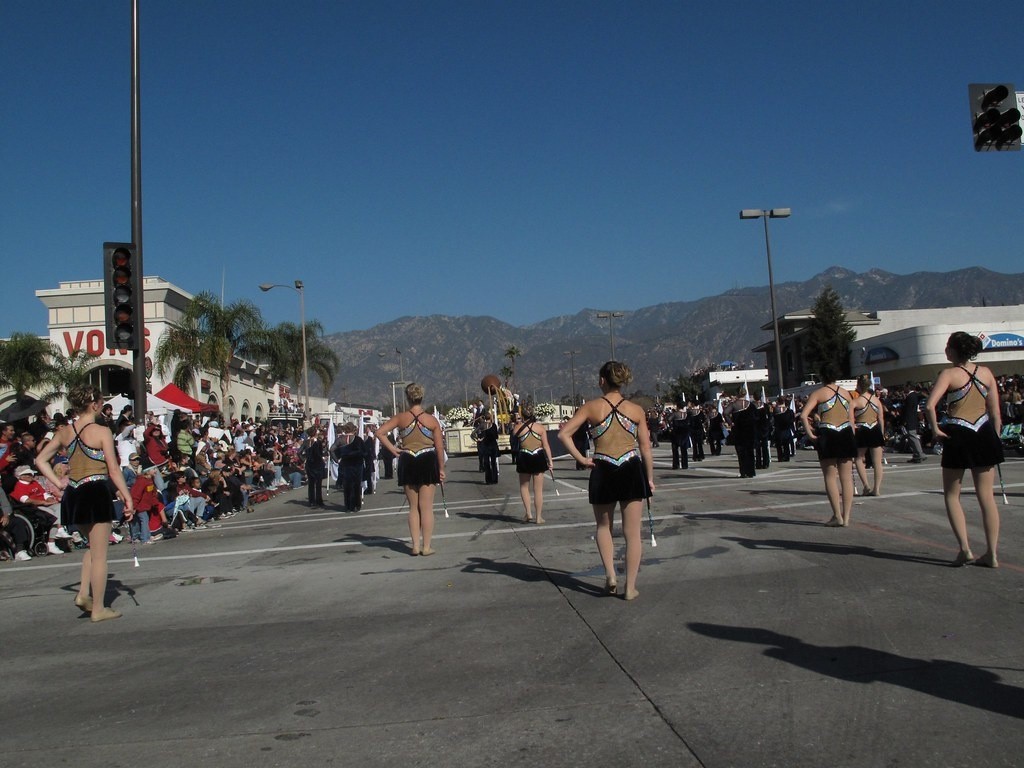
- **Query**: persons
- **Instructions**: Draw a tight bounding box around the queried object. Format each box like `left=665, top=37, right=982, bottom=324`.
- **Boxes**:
left=852, top=374, right=884, bottom=496
left=296, top=427, right=327, bottom=508
left=36, top=386, right=134, bottom=621
left=642, top=367, right=1024, bottom=470
left=557, top=361, right=655, bottom=600
left=668, top=401, right=689, bottom=470
left=0, top=386, right=399, bottom=562
left=330, top=422, right=369, bottom=515
left=468, top=394, right=502, bottom=485
left=926, top=331, right=1006, bottom=567
left=723, top=388, right=759, bottom=478
left=374, top=383, right=446, bottom=556
left=512, top=404, right=554, bottom=524
left=800, top=368, right=851, bottom=527
left=899, top=381, right=928, bottom=463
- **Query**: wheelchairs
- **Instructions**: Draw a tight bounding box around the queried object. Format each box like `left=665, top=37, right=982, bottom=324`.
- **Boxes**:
left=13, top=504, right=58, bottom=557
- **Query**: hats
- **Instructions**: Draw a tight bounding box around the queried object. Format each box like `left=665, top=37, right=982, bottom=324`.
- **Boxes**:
left=13, top=465, right=34, bottom=480
left=129, top=453, right=141, bottom=460
left=223, top=466, right=231, bottom=471
left=143, top=461, right=155, bottom=472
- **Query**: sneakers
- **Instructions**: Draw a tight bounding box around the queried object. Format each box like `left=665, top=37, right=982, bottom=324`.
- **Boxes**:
left=71, top=532, right=83, bottom=543
left=55, top=527, right=71, bottom=538
left=47, top=542, right=64, bottom=554
left=15, top=550, right=31, bottom=560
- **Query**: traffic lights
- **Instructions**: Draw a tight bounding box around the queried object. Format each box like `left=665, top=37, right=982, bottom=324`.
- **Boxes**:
left=103, top=241, right=139, bottom=349
left=969, top=82, right=1022, bottom=152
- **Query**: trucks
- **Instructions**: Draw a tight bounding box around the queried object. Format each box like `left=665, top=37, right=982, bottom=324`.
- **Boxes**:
left=267, top=412, right=303, bottom=429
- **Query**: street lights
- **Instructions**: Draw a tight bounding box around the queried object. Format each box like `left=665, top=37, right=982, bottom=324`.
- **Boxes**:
left=396, top=347, right=405, bottom=412
left=739, top=208, right=790, bottom=395
left=597, top=312, right=624, bottom=361
left=563, top=351, right=581, bottom=414
left=258, top=280, right=311, bottom=430
left=390, top=382, right=396, bottom=441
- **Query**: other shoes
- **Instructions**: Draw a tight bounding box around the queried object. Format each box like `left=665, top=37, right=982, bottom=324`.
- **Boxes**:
left=952, top=548, right=974, bottom=567
left=536, top=518, right=545, bottom=524
left=862, top=485, right=871, bottom=494
left=91, top=607, right=122, bottom=621
left=423, top=549, right=435, bottom=556
left=74, top=594, right=93, bottom=612
left=908, top=457, right=927, bottom=464
left=824, top=515, right=849, bottom=527
left=976, top=553, right=998, bottom=567
left=110, top=505, right=246, bottom=544
left=605, top=578, right=617, bottom=595
left=624, top=587, right=639, bottom=600
left=869, top=490, right=878, bottom=496
left=523, top=514, right=533, bottom=522
left=411, top=548, right=420, bottom=555
